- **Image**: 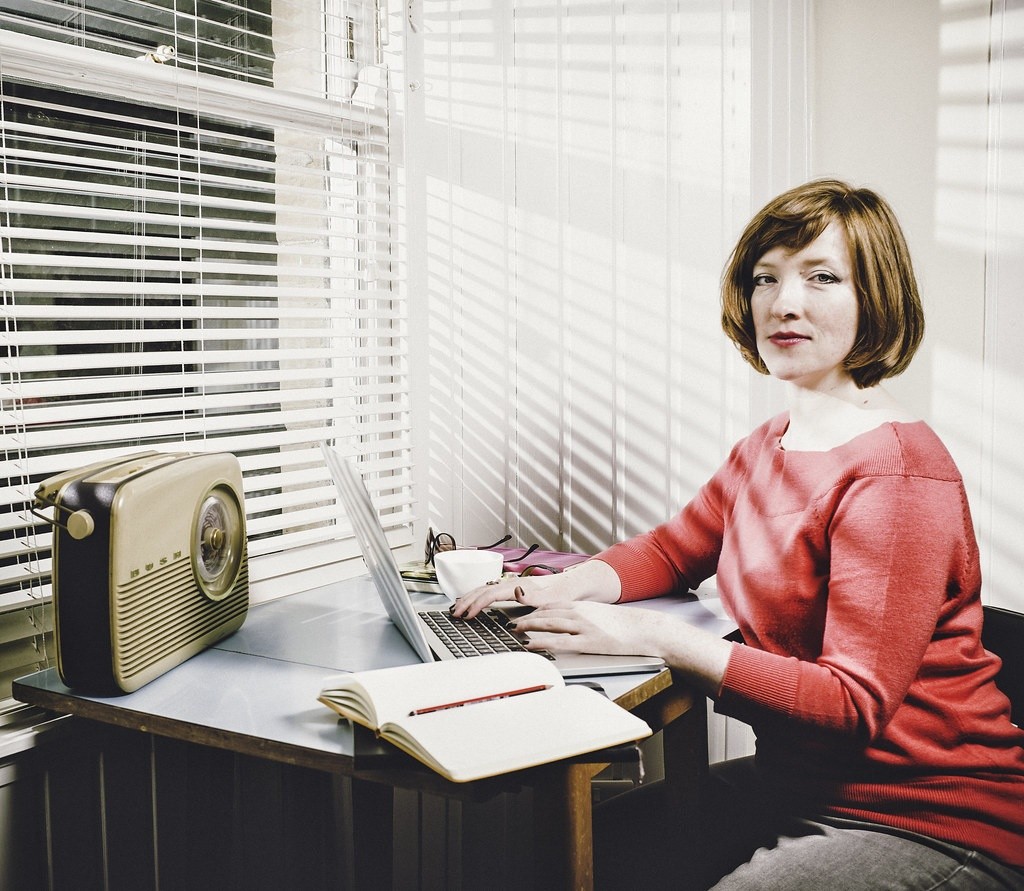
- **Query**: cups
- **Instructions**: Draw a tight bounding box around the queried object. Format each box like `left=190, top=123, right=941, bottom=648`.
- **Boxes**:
left=434, top=549, right=504, bottom=603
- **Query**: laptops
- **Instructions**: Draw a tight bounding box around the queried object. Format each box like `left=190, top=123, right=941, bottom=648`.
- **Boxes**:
left=318, top=441, right=666, bottom=677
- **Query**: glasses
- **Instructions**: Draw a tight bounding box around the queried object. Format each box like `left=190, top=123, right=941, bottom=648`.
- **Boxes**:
left=424, top=526, right=540, bottom=569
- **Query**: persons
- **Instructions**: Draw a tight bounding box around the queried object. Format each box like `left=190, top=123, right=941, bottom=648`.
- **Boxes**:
left=448, top=180, right=1024, bottom=891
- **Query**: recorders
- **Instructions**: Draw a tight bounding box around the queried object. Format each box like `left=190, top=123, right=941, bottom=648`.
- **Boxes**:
left=50, top=451, right=249, bottom=698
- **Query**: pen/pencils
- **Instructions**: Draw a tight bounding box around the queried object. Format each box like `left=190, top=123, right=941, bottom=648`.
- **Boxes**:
left=408, top=683, right=556, bottom=716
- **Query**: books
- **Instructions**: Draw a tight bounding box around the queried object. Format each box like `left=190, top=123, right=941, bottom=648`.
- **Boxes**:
left=400, top=568, right=443, bottom=594
left=318, top=652, right=653, bottom=783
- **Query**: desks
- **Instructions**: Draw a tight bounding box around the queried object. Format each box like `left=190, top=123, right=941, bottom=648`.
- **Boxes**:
left=11, top=534, right=741, bottom=891
